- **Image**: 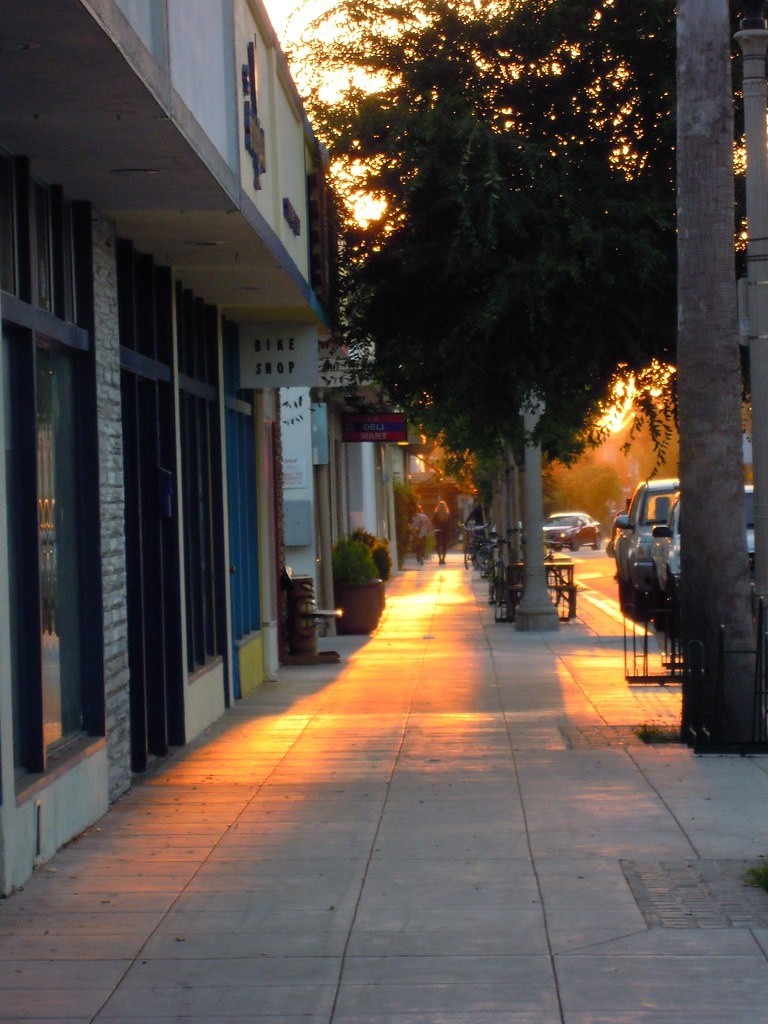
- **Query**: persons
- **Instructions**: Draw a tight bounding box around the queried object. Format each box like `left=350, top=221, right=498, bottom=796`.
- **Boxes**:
left=465, top=498, right=491, bottom=538
left=411, top=506, right=429, bottom=564
left=610, top=498, right=632, bottom=580
left=431, top=501, right=449, bottom=565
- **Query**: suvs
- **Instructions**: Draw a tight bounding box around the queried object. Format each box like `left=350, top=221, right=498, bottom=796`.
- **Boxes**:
left=611, top=479, right=685, bottom=621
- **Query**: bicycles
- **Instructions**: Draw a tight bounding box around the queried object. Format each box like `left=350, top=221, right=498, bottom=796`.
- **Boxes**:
left=460, top=522, right=521, bottom=586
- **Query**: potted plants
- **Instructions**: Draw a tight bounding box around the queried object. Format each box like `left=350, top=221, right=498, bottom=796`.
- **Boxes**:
left=332, top=535, right=382, bottom=635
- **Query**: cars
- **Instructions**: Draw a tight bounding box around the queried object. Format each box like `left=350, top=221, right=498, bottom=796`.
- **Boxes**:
left=542, top=512, right=603, bottom=552
left=649, top=483, right=756, bottom=631
